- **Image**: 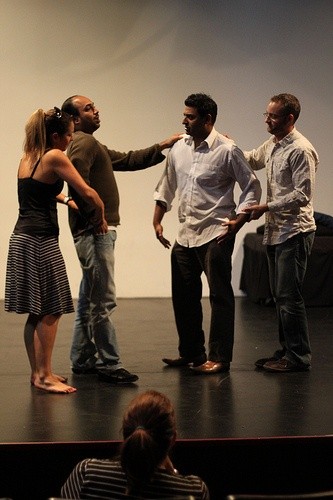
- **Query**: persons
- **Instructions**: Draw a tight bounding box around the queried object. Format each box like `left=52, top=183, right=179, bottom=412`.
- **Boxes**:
left=4, top=107, right=108, bottom=394
left=60, top=390, right=209, bottom=500
left=153, top=94, right=262, bottom=373
left=61, top=95, right=184, bottom=383
left=224, top=94, right=319, bottom=373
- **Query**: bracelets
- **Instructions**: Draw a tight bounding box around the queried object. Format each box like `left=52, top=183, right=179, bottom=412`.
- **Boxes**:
left=64, top=196, right=72, bottom=204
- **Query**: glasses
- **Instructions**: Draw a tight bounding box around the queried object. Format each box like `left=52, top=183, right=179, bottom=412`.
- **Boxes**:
left=263, top=112, right=280, bottom=120
left=54, top=106, right=62, bottom=131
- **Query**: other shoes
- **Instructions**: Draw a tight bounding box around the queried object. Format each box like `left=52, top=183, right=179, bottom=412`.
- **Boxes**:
left=163, top=354, right=208, bottom=367
left=71, top=366, right=96, bottom=376
left=96, top=368, right=139, bottom=383
left=254, top=350, right=312, bottom=372
left=187, top=360, right=230, bottom=374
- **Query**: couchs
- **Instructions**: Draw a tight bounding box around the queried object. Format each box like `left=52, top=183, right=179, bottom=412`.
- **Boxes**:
left=240, top=212, right=333, bottom=308
